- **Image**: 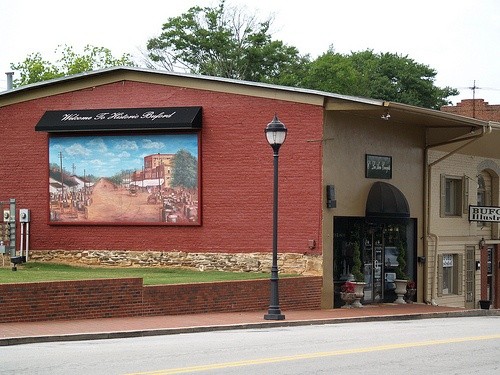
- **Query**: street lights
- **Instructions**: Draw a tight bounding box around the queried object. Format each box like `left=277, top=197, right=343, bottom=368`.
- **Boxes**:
left=264, top=114, right=288, bottom=320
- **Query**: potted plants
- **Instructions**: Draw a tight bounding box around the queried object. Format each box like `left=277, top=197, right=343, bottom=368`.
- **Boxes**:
left=392, top=241, right=408, bottom=303
left=350, top=243, right=365, bottom=307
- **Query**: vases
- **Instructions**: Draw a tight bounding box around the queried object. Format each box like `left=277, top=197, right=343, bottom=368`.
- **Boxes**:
left=341, top=293, right=356, bottom=309
left=406, top=289, right=416, bottom=303
left=479, top=300, right=492, bottom=310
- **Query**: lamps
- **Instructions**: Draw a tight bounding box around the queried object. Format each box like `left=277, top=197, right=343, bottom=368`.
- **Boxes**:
left=381, top=108, right=391, bottom=120
left=326, top=184, right=336, bottom=208
left=11, top=255, right=21, bottom=271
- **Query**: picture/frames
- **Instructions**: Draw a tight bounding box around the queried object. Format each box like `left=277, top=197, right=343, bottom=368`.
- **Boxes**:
left=366, top=154, right=391, bottom=180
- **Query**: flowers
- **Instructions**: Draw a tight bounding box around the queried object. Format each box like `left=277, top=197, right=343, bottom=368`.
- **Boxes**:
left=406, top=279, right=416, bottom=289
left=340, top=281, right=355, bottom=292
